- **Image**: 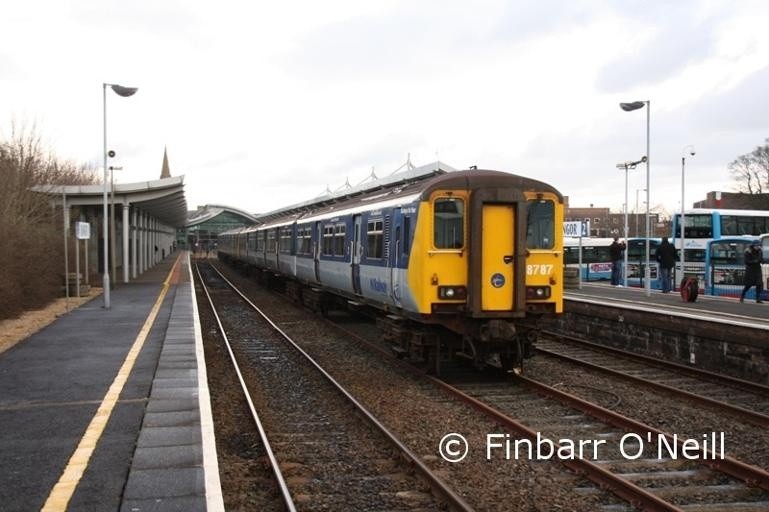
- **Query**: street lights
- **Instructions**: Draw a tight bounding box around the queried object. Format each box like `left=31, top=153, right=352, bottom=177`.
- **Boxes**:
left=103, top=83, right=140, bottom=310
left=679, top=144, right=696, bottom=288
left=616, top=98, right=651, bottom=298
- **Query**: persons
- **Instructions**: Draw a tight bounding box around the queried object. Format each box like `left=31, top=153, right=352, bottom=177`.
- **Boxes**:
left=655, top=235, right=680, bottom=294
left=739, top=240, right=769, bottom=304
left=609, top=236, right=626, bottom=288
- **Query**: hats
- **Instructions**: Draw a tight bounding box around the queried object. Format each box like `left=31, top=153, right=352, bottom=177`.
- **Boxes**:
left=750, top=239, right=762, bottom=248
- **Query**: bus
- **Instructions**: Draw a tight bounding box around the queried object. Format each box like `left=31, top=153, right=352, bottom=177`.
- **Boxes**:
left=563, top=209, right=769, bottom=303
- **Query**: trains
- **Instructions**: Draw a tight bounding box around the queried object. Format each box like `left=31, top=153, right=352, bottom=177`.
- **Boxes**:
left=217, top=169, right=565, bottom=377
